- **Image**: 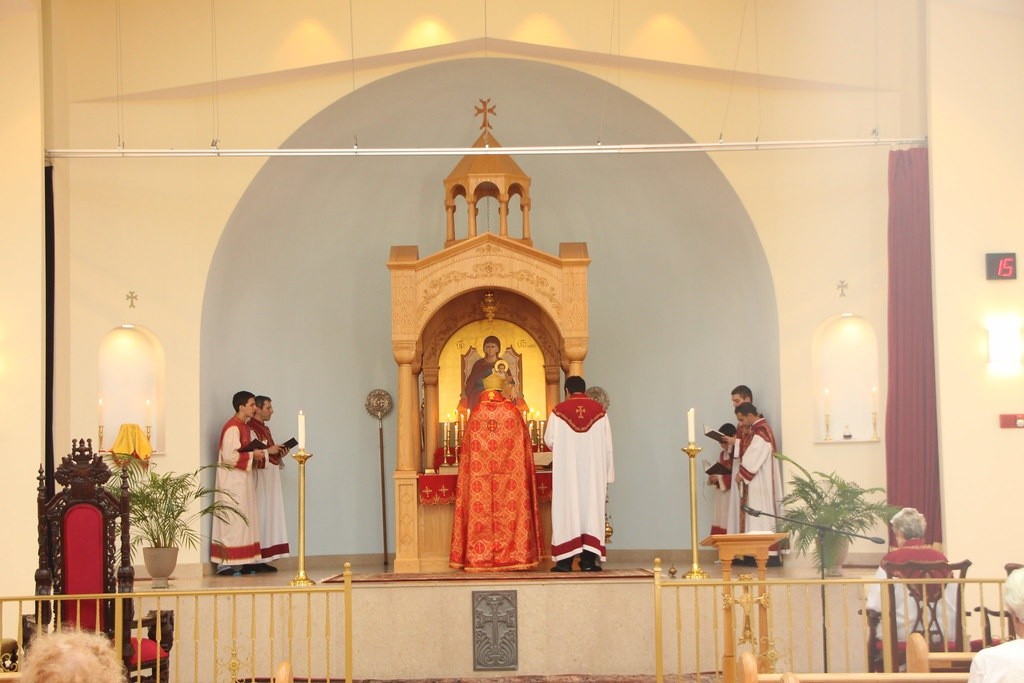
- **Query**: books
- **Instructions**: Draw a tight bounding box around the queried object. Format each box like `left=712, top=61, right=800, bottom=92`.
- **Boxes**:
left=701, top=456, right=732, bottom=476
left=702, top=423, right=727, bottom=444
left=240, top=438, right=268, bottom=452
left=277, top=437, right=298, bottom=456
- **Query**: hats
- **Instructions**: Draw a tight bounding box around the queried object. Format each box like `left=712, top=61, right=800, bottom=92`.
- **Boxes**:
left=482, top=373, right=505, bottom=392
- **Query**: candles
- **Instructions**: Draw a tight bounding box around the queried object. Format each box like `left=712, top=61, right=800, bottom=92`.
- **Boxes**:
left=298, top=410, right=306, bottom=452
left=444, top=408, right=545, bottom=449
left=688, top=408, right=696, bottom=444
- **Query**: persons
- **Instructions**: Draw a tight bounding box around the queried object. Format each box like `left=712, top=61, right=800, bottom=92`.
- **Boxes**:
left=734, top=403, right=787, bottom=531
left=709, top=422, right=736, bottom=535
left=209, top=393, right=265, bottom=577
left=448, top=372, right=546, bottom=572
left=14, top=622, right=130, bottom=683
left=543, top=377, right=615, bottom=573
left=967, top=568, right=1024, bottom=683
left=495, top=362, right=521, bottom=401
left=246, top=396, right=290, bottom=574
left=864, top=508, right=958, bottom=658
left=464, top=338, right=514, bottom=409
left=721, top=388, right=764, bottom=533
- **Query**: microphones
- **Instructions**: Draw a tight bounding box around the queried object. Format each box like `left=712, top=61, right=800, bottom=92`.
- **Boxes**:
left=740, top=504, right=759, bottom=517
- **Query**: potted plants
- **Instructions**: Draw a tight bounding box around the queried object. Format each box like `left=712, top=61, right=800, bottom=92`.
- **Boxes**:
left=768, top=451, right=905, bottom=575
left=100, top=453, right=252, bottom=586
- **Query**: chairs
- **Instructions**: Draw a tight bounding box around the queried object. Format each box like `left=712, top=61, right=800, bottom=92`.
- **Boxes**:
left=856, top=560, right=973, bottom=674
left=17, top=438, right=176, bottom=683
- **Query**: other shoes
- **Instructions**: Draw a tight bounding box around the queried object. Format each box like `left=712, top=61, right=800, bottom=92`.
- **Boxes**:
left=734, top=557, right=758, bottom=567
left=766, top=556, right=783, bottom=566
left=220, top=568, right=234, bottom=576
left=551, top=566, right=572, bottom=572
left=254, top=563, right=278, bottom=573
left=579, top=563, right=602, bottom=571
left=240, top=565, right=257, bottom=574
